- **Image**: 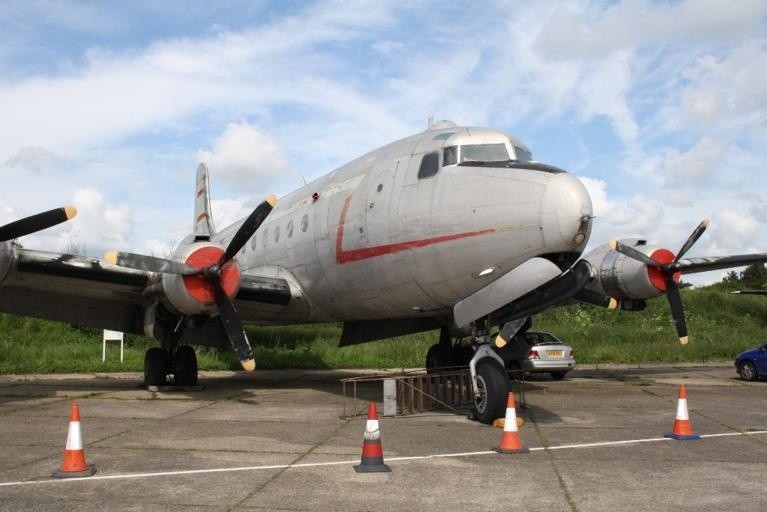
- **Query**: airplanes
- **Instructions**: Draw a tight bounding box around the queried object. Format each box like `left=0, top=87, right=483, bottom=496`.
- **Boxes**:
left=2, top=120, right=767, bottom=424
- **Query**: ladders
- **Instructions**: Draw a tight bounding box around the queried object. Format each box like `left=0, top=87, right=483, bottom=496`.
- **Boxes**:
left=339, top=365, right=527, bottom=420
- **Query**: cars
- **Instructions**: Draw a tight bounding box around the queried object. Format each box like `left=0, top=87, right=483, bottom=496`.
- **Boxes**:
left=492, top=331, right=575, bottom=381
left=734, top=342, right=766, bottom=382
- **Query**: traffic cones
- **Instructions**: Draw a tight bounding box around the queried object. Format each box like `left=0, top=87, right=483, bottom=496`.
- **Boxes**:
left=491, top=391, right=530, bottom=453
left=665, top=386, right=699, bottom=441
left=352, top=401, right=391, bottom=474
left=53, top=403, right=96, bottom=478
